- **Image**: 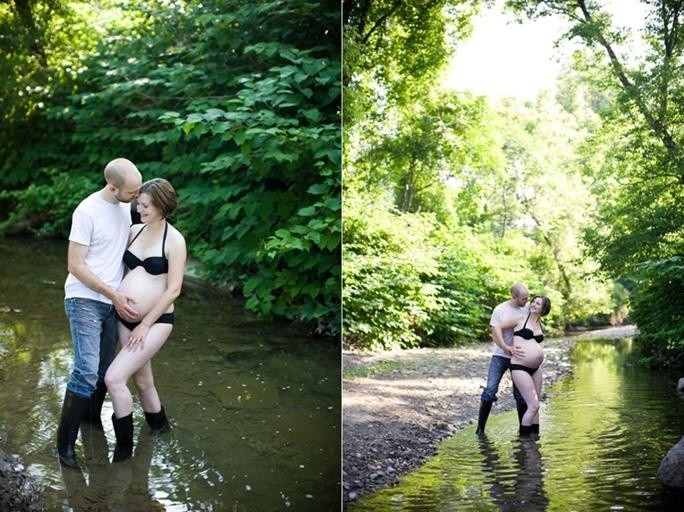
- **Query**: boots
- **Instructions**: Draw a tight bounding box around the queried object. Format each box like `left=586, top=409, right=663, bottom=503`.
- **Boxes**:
left=515, top=402, right=542, bottom=439
left=143, top=405, right=173, bottom=437
left=111, top=411, right=134, bottom=464
left=475, top=401, right=493, bottom=435
left=83, top=381, right=107, bottom=431
left=57, top=388, right=89, bottom=472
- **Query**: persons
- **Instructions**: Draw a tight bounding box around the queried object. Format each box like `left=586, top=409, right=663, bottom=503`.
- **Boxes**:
left=475, top=283, right=531, bottom=436
left=519, top=438, right=547, bottom=512
left=55, top=157, right=143, bottom=474
left=102, top=176, right=189, bottom=462
left=477, top=432, right=539, bottom=512
left=493, top=295, right=552, bottom=438
